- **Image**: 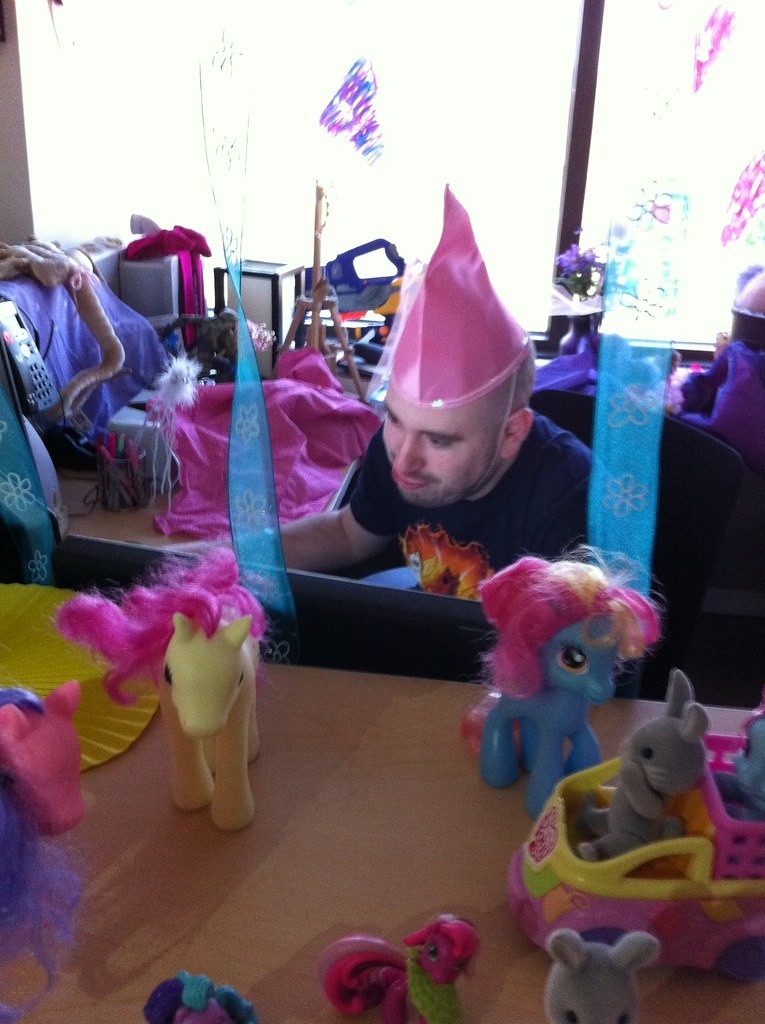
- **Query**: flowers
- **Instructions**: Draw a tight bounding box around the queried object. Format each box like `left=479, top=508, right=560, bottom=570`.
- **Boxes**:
left=551, top=225, right=608, bottom=305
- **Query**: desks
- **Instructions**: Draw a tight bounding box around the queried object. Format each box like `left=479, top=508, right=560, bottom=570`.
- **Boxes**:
left=0, top=655, right=765, bottom=1024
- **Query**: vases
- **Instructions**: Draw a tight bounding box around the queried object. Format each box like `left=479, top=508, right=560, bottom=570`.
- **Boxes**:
left=558, top=310, right=601, bottom=395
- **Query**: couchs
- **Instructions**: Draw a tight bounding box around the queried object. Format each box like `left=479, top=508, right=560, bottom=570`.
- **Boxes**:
left=1, top=237, right=191, bottom=483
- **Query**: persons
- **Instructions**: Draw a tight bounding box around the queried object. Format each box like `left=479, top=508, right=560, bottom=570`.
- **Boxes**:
left=156, top=324, right=664, bottom=628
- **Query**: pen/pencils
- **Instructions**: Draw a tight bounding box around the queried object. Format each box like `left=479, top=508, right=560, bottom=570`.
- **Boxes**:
left=93, top=428, right=148, bottom=512
left=0, top=301, right=62, bottom=418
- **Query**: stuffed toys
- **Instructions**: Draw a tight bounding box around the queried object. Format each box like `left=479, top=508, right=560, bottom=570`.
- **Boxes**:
left=0, top=536, right=764, bottom=1024
left=660, top=265, right=765, bottom=467
left=0, top=226, right=126, bottom=422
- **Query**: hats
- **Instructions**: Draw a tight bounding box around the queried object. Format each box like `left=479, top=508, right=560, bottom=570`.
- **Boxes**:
left=389, top=185, right=530, bottom=407
